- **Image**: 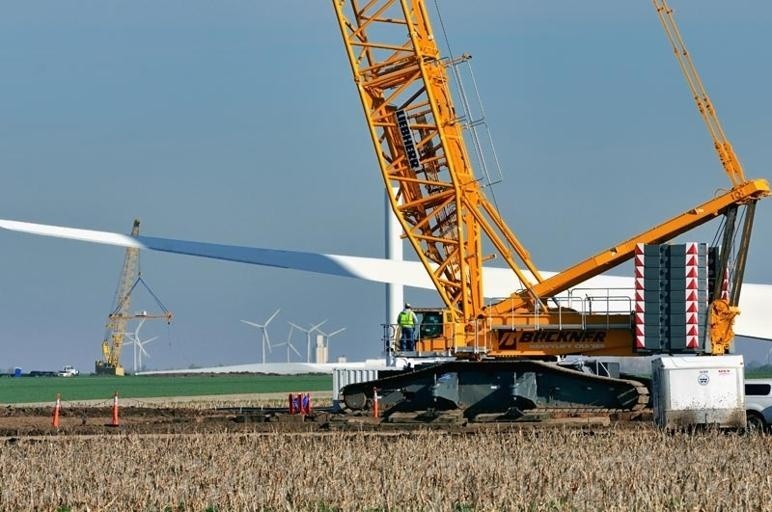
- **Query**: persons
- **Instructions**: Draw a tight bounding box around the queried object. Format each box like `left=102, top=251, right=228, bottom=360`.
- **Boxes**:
left=395, top=303, right=419, bottom=352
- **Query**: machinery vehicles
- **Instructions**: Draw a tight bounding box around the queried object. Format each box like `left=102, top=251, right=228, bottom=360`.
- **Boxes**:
left=95, top=219, right=172, bottom=376
left=334, top=0, right=772, bottom=416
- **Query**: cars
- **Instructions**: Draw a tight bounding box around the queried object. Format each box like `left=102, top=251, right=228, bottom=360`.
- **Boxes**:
left=744, top=378, right=772, bottom=432
left=60, top=366, right=79, bottom=377
